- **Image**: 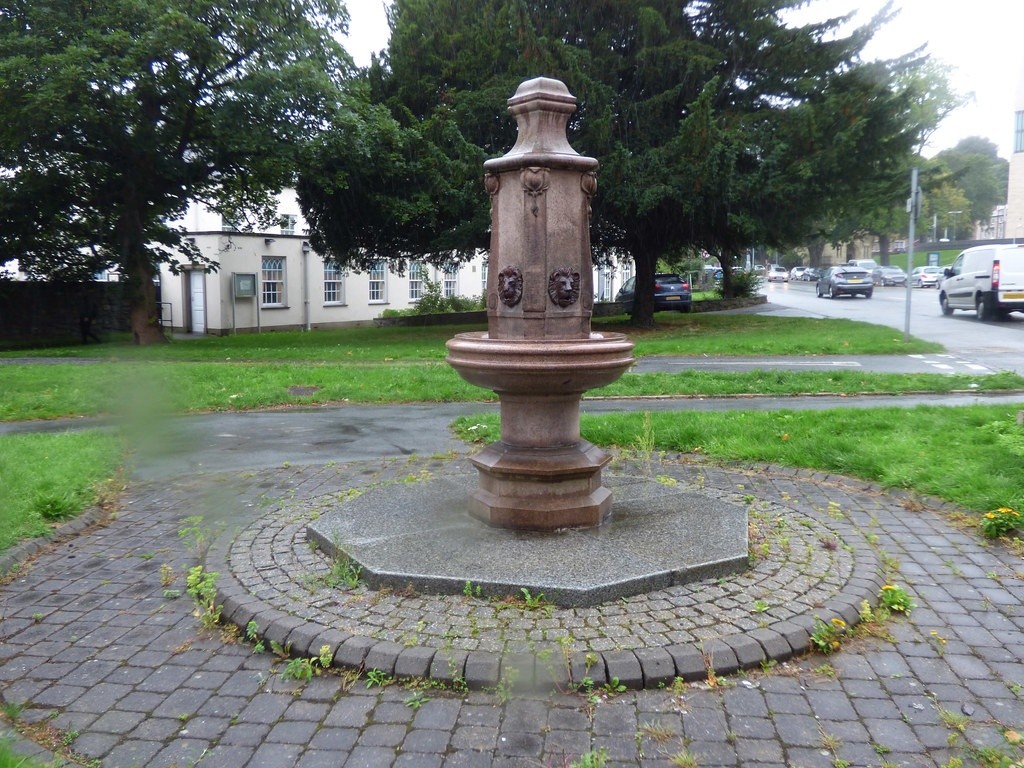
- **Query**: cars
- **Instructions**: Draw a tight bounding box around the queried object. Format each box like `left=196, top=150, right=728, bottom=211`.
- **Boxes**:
left=615, top=273, right=692, bottom=313
left=767, top=267, right=789, bottom=283
left=790, top=267, right=823, bottom=281
left=816, top=266, right=874, bottom=299
left=911, top=264, right=953, bottom=289
left=755, top=265, right=766, bottom=275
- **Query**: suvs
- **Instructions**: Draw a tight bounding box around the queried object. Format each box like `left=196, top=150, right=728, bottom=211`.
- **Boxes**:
left=873, top=265, right=908, bottom=287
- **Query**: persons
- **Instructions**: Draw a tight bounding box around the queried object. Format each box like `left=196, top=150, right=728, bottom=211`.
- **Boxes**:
left=74, top=287, right=101, bottom=344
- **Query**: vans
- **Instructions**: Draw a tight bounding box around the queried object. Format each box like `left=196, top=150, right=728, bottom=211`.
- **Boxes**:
left=848, top=259, right=878, bottom=274
left=939, top=244, right=1024, bottom=321
